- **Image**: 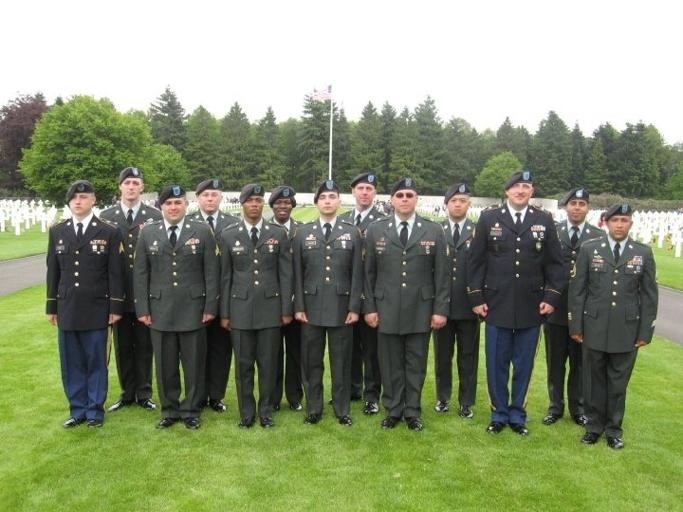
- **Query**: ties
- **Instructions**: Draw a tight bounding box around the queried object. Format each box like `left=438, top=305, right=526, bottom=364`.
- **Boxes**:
left=613, top=243, right=619, bottom=262
left=514, top=212, right=520, bottom=233
left=356, top=213, right=360, bottom=225
left=399, top=222, right=408, bottom=245
left=250, top=227, right=257, bottom=245
left=323, top=223, right=331, bottom=240
left=206, top=215, right=214, bottom=233
left=570, top=226, right=578, bottom=246
left=77, top=210, right=179, bottom=249
left=453, top=223, right=459, bottom=243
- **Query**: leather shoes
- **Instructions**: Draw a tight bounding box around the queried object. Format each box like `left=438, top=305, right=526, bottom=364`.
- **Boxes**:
left=459, top=405, right=473, bottom=419
left=63, top=417, right=86, bottom=428
left=156, top=417, right=177, bottom=429
left=137, top=399, right=155, bottom=410
left=328, top=396, right=422, bottom=432
left=433, top=401, right=448, bottom=413
left=180, top=417, right=199, bottom=429
left=238, top=401, right=321, bottom=429
left=507, top=418, right=528, bottom=436
left=486, top=421, right=505, bottom=434
left=543, top=413, right=624, bottom=450
left=209, top=399, right=226, bottom=411
left=107, top=400, right=125, bottom=412
left=88, top=419, right=102, bottom=428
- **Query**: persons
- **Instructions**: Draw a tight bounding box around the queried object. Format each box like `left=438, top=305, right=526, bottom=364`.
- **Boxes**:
left=131, top=185, right=221, bottom=431
left=44, top=180, right=128, bottom=428
left=219, top=182, right=294, bottom=430
left=567, top=203, right=659, bottom=450
left=432, top=182, right=481, bottom=421
left=361, top=176, right=451, bottom=431
left=293, top=179, right=364, bottom=425
left=185, top=177, right=242, bottom=413
left=329, top=169, right=387, bottom=416
left=98, top=167, right=163, bottom=413
left=541, top=186, right=606, bottom=429
left=266, top=185, right=306, bottom=413
left=463, top=170, right=567, bottom=438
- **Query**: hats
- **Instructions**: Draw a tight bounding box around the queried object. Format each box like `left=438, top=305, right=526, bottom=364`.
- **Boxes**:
left=443, top=182, right=470, bottom=203
left=350, top=172, right=377, bottom=187
left=560, top=187, right=588, bottom=205
left=390, top=178, right=415, bottom=196
left=504, top=171, right=532, bottom=190
left=65, top=180, right=94, bottom=204
left=159, top=185, right=185, bottom=205
left=195, top=179, right=295, bottom=208
left=119, top=167, right=143, bottom=184
left=314, top=180, right=339, bottom=203
left=604, top=203, right=631, bottom=221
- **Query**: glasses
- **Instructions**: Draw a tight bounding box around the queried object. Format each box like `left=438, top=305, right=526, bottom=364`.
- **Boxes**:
left=395, top=192, right=413, bottom=198
